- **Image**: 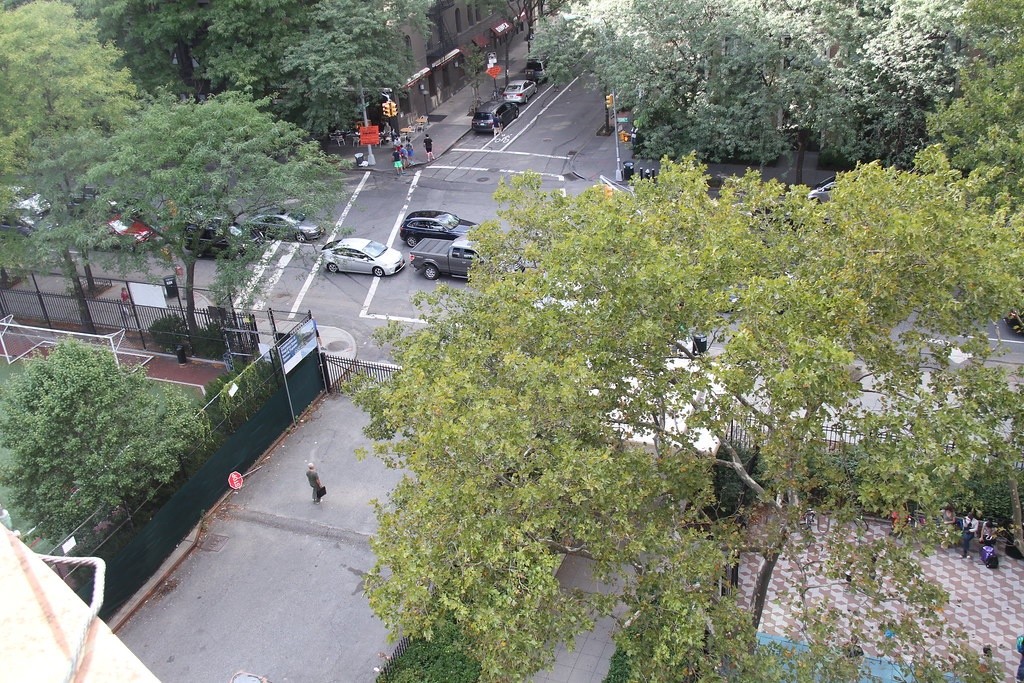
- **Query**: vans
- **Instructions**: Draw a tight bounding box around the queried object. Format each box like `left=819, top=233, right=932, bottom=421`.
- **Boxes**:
left=470, top=98, right=519, bottom=134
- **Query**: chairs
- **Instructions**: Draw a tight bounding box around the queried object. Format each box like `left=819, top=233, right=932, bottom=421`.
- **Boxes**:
left=351, top=137, right=360, bottom=147
left=419, top=115, right=428, bottom=119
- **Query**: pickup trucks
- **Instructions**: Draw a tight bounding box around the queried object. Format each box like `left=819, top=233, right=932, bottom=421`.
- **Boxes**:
left=410, top=235, right=526, bottom=279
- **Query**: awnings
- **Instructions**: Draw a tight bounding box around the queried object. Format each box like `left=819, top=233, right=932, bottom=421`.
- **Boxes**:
left=472, top=32, right=489, bottom=50
left=516, top=9, right=530, bottom=22
left=488, top=19, right=513, bottom=37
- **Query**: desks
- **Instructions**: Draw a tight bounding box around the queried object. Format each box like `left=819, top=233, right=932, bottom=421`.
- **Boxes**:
left=400, top=127, right=411, bottom=139
left=416, top=118, right=427, bottom=125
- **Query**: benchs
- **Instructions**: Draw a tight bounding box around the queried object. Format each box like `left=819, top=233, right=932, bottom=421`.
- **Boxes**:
left=914, top=510, right=998, bottom=547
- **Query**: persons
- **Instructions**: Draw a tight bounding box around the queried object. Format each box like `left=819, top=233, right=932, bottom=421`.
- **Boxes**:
left=422, top=134, right=436, bottom=162
left=492, top=113, right=503, bottom=141
left=120, top=287, right=134, bottom=315
left=306, top=463, right=322, bottom=504
left=381, top=121, right=413, bottom=176
left=890, top=505, right=998, bottom=560
left=975, top=645, right=996, bottom=674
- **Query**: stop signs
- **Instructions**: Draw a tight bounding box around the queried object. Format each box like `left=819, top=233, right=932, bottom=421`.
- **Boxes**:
left=229, top=470, right=243, bottom=489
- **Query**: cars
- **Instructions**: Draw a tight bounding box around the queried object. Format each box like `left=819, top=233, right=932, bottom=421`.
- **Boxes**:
left=502, top=79, right=538, bottom=104
left=106, top=213, right=156, bottom=252
left=809, top=174, right=845, bottom=203
left=65, top=186, right=145, bottom=220
left=1, top=209, right=54, bottom=241
left=1004, top=308, right=1024, bottom=335
left=7, top=186, right=50, bottom=220
left=320, top=238, right=405, bottom=277
left=249, top=208, right=326, bottom=242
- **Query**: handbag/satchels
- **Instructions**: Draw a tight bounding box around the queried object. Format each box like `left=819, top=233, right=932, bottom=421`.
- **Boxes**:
left=317, top=486, right=326, bottom=498
left=121, top=307, right=129, bottom=316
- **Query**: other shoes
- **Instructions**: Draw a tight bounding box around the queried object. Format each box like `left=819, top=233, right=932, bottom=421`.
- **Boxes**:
left=428, top=160, right=431, bottom=163
left=402, top=172, right=406, bottom=174
left=432, top=157, right=436, bottom=160
left=397, top=173, right=400, bottom=175
left=313, top=499, right=322, bottom=503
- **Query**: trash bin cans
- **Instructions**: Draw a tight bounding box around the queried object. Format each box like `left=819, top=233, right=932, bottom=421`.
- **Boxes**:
left=692, top=334, right=708, bottom=356
left=355, top=153, right=364, bottom=166
left=981, top=546, right=999, bottom=569
left=162, top=274, right=181, bottom=298
left=622, top=161, right=635, bottom=180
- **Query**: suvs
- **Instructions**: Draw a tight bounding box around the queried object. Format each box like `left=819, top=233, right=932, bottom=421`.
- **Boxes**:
left=400, top=209, right=482, bottom=248
left=525, top=54, right=551, bottom=80
left=184, top=218, right=265, bottom=260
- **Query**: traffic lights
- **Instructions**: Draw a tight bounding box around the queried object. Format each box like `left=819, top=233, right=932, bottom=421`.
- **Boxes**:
left=623, top=134, right=631, bottom=142
left=382, top=103, right=390, bottom=117
left=390, top=102, right=399, bottom=117
left=606, top=94, right=613, bottom=110
